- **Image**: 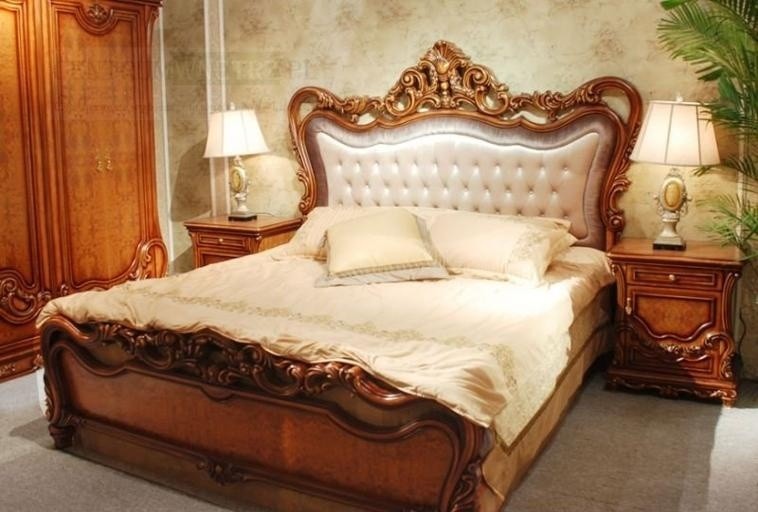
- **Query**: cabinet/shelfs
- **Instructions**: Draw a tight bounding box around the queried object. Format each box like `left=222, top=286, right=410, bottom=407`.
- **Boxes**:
left=0, top=2, right=168, bottom=383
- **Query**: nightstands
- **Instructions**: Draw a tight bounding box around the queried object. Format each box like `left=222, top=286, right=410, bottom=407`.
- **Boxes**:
left=602, top=233, right=748, bottom=405
left=182, top=210, right=304, bottom=271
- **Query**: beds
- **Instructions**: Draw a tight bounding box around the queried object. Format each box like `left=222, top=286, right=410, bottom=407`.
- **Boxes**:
left=35, top=38, right=644, bottom=511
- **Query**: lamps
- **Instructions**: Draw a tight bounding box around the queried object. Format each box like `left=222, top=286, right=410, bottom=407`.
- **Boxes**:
left=202, top=108, right=271, bottom=222
left=628, top=98, right=724, bottom=252
